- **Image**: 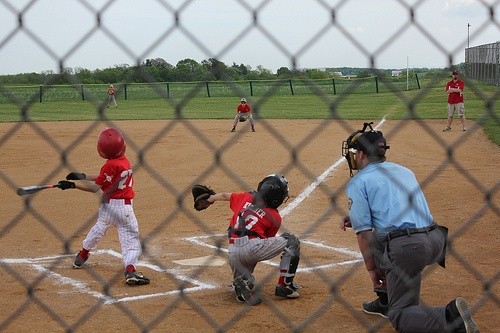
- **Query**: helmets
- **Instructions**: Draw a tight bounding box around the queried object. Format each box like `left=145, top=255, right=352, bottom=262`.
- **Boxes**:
left=110, top=85, right=113, bottom=88
left=97, top=128, right=127, bottom=160
left=257, top=173, right=289, bottom=203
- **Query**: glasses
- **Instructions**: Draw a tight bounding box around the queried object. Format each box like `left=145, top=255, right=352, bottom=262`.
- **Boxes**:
left=242, top=102, right=246, bottom=104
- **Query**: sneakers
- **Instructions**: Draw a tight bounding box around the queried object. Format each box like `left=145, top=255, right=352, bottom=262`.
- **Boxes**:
left=445, top=296, right=480, bottom=333
left=362, top=298, right=390, bottom=318
left=125, top=271, right=150, bottom=285
left=443, top=126, right=451, bottom=132
left=276, top=285, right=300, bottom=298
left=73, top=253, right=84, bottom=268
left=463, top=126, right=466, bottom=131
left=234, top=278, right=261, bottom=304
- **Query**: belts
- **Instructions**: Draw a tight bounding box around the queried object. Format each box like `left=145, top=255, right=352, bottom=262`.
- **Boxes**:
left=229, top=236, right=257, bottom=244
left=106, top=199, right=131, bottom=204
left=385, top=222, right=437, bottom=242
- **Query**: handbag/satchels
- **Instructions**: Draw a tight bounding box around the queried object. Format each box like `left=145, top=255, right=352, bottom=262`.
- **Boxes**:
left=437, top=224, right=448, bottom=268
left=380, top=241, right=391, bottom=269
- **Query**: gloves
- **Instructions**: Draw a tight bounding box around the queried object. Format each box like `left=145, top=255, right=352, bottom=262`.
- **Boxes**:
left=66, top=172, right=87, bottom=180
left=58, top=181, right=75, bottom=190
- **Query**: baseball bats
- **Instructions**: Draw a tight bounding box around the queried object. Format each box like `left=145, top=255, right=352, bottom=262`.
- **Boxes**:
left=17, top=183, right=61, bottom=196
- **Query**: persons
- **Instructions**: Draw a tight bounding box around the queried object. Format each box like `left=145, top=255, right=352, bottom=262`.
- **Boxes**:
left=230, top=98, right=256, bottom=132
left=191, top=173, right=302, bottom=306
left=339, top=120, right=480, bottom=333
left=443, top=69, right=467, bottom=132
left=106, top=84, right=117, bottom=108
left=56, top=128, right=151, bottom=286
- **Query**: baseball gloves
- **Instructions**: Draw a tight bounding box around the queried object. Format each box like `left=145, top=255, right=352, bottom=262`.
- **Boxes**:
left=239, top=117, right=246, bottom=122
left=192, top=184, right=218, bottom=211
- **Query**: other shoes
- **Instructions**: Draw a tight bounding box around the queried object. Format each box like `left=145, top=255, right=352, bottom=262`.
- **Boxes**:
left=251, top=128, right=255, bottom=132
left=231, top=128, right=235, bottom=132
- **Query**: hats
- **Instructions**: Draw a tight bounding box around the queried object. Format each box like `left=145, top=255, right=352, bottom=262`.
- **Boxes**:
left=352, top=131, right=386, bottom=156
left=452, top=69, right=458, bottom=74
left=241, top=98, right=246, bottom=102
